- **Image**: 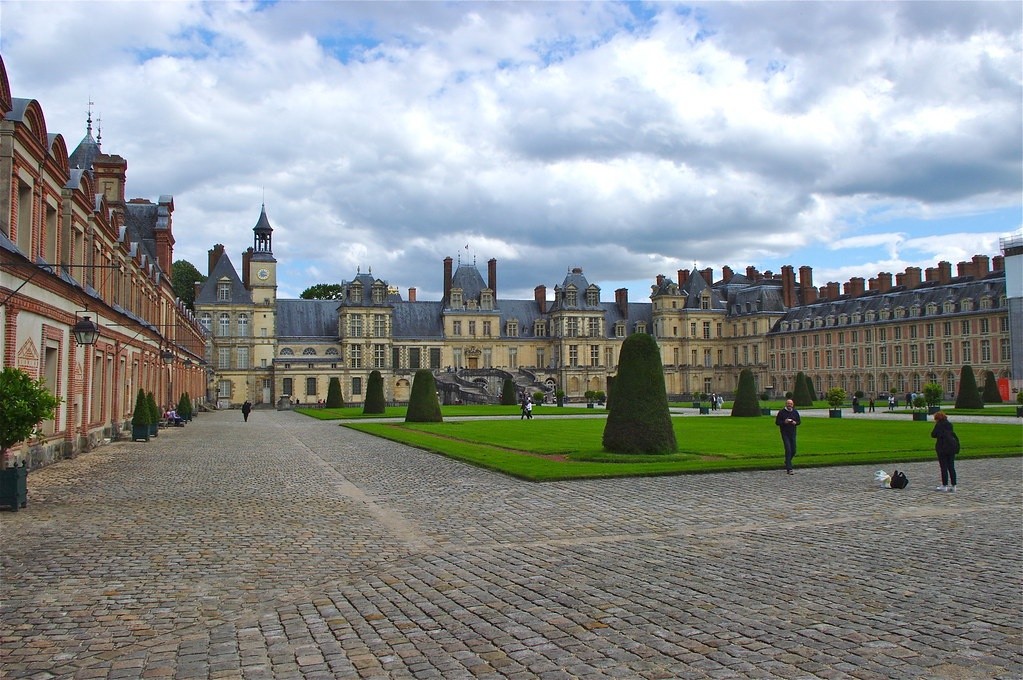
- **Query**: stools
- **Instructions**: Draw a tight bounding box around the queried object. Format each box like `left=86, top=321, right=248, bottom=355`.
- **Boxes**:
left=159, top=418, right=168, bottom=429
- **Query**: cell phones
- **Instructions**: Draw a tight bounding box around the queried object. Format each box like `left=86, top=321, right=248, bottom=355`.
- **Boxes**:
left=789, top=421, right=793, bottom=422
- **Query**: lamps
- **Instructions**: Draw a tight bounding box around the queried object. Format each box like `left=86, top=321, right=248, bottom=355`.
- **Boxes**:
left=0, top=263, right=121, bottom=348
left=144, top=338, right=201, bottom=369
left=197, top=360, right=208, bottom=368
left=209, top=371, right=223, bottom=382
left=106, top=324, right=182, bottom=364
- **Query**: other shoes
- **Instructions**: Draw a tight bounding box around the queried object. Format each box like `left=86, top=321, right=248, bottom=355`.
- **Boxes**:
left=787, top=469, right=794, bottom=475
left=937, top=485, right=947, bottom=492
left=947, top=485, right=956, bottom=493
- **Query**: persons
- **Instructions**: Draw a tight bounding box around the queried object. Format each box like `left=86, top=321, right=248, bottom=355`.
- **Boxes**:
left=709, top=393, right=724, bottom=411
left=165, top=408, right=184, bottom=427
left=852, top=396, right=857, bottom=413
left=775, top=399, right=801, bottom=475
left=296, top=398, right=299, bottom=404
left=520, top=398, right=534, bottom=419
left=318, top=399, right=325, bottom=408
left=242, top=402, right=252, bottom=422
left=869, top=392, right=924, bottom=412
left=931, top=411, right=957, bottom=493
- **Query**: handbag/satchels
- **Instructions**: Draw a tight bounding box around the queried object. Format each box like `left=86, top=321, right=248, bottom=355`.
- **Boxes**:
left=875, top=470, right=891, bottom=488
left=952, top=432, right=960, bottom=455
left=890, top=472, right=908, bottom=490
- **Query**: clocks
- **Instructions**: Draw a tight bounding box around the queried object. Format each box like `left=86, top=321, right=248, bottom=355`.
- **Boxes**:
left=256, top=267, right=270, bottom=280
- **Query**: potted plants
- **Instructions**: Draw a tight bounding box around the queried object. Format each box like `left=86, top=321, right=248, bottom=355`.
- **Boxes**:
left=533, top=392, right=544, bottom=406
left=853, top=390, right=865, bottom=413
left=912, top=395, right=928, bottom=421
left=923, top=379, right=944, bottom=415
left=175, top=393, right=189, bottom=424
left=556, top=389, right=565, bottom=407
left=1016, top=390, right=1023, bottom=418
left=693, top=392, right=701, bottom=409
left=594, top=390, right=606, bottom=405
left=759, top=393, right=770, bottom=415
left=890, top=387, right=899, bottom=407
left=827, top=386, right=846, bottom=418
left=0, top=366, right=66, bottom=512
left=146, top=391, right=160, bottom=438
left=699, top=394, right=709, bottom=414
left=131, top=388, right=152, bottom=442
left=584, top=390, right=596, bottom=408
left=185, top=392, right=193, bottom=421
